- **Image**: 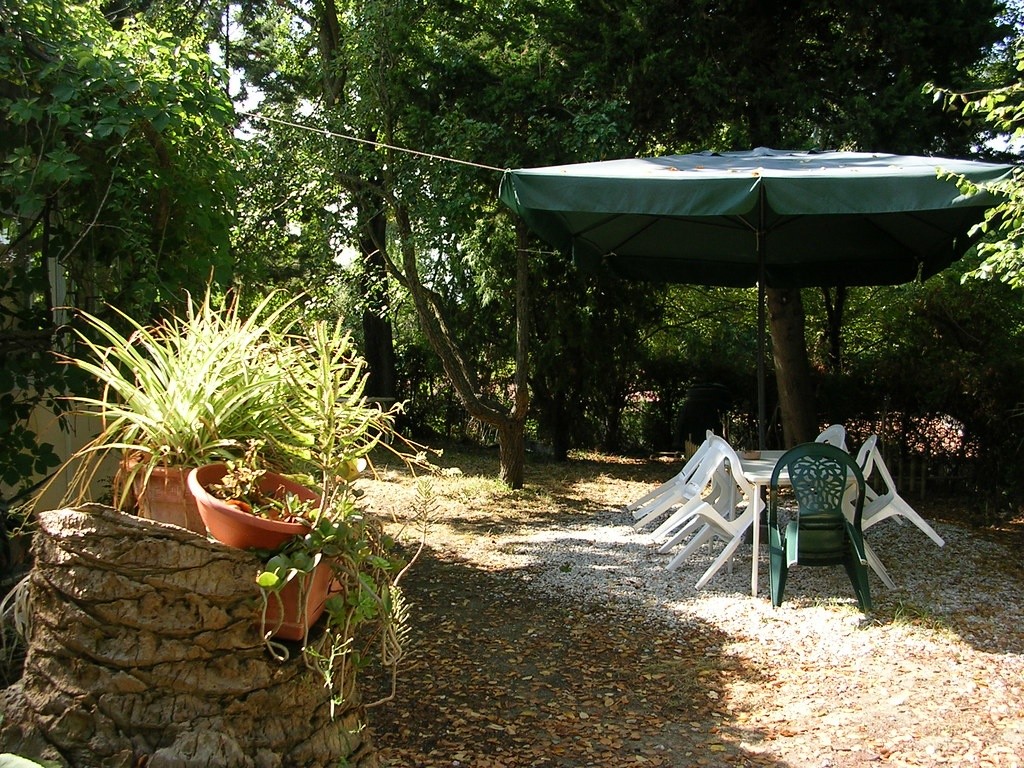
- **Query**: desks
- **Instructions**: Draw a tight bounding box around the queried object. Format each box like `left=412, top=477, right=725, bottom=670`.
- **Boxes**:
left=735, top=450, right=867, bottom=596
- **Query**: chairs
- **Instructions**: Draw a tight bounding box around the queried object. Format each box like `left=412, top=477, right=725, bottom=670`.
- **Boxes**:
left=627, top=430, right=743, bottom=553
left=768, top=442, right=872, bottom=613
left=815, top=424, right=903, bottom=527
left=841, top=435, right=944, bottom=590
left=634, top=435, right=766, bottom=591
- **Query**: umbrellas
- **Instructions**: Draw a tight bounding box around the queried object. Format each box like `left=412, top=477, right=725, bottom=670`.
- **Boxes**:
left=498, top=145, right=1023, bottom=442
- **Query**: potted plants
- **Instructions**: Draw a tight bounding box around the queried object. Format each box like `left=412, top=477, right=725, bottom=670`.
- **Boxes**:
left=23, top=264, right=393, bottom=536
left=189, top=437, right=322, bottom=551
left=256, top=476, right=438, bottom=721
